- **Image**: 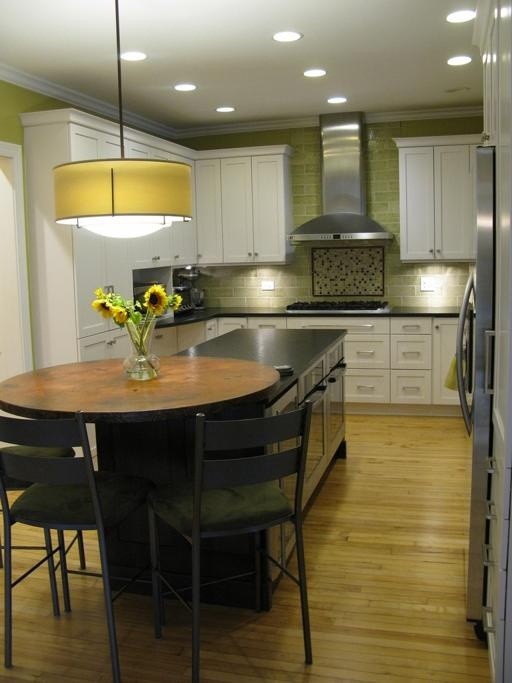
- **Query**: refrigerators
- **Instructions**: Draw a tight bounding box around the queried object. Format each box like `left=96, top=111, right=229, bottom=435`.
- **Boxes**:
left=456, top=144, right=497, bottom=644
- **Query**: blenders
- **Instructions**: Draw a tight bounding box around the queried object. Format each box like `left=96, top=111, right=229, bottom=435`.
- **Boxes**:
left=190, top=286, right=206, bottom=310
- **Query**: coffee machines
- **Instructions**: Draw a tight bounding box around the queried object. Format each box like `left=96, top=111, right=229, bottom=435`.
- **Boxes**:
left=171, top=269, right=202, bottom=316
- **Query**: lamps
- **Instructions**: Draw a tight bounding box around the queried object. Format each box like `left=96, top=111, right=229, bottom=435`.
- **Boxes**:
left=53, top=0, right=196, bottom=241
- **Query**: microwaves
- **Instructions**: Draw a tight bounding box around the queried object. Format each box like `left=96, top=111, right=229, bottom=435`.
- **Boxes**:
left=133, top=282, right=167, bottom=315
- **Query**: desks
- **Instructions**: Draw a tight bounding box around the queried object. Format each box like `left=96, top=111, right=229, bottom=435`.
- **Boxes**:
left=0, top=352, right=282, bottom=641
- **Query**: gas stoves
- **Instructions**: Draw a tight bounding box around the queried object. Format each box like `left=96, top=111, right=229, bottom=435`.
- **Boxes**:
left=284, top=298, right=390, bottom=315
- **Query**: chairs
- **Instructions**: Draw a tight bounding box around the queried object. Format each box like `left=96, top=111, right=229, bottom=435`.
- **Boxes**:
left=146, top=405, right=315, bottom=679
left=0, top=444, right=86, bottom=616
left=0, top=415, right=136, bottom=681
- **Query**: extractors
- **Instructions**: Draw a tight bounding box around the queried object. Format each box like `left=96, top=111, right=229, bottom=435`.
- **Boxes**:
left=287, top=111, right=394, bottom=241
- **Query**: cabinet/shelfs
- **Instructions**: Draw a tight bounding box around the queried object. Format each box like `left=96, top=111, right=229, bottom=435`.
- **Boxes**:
left=194, top=144, right=295, bottom=266
left=56, top=307, right=486, bottom=411
left=21, top=106, right=194, bottom=337
left=392, top=134, right=478, bottom=265
